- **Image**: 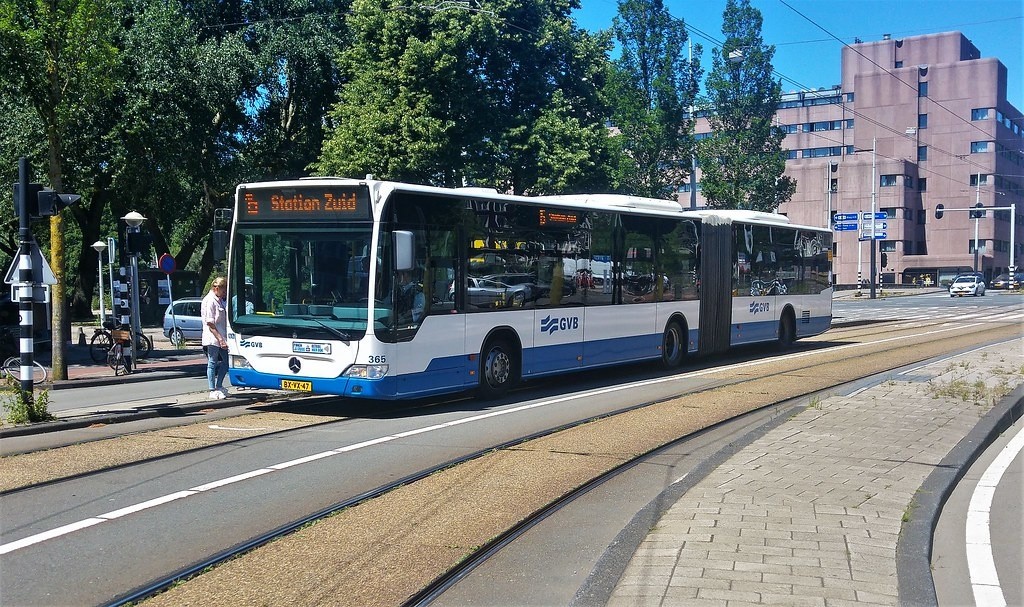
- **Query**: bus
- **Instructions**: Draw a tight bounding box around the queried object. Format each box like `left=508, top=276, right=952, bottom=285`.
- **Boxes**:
left=210, top=171, right=833, bottom=402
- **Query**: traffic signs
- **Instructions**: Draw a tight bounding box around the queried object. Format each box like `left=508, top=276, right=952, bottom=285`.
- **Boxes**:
left=862, top=212, right=888, bottom=220
left=861, top=231, right=887, bottom=239
left=833, top=213, right=858, bottom=222
left=862, top=222, right=888, bottom=230
left=833, top=223, right=857, bottom=231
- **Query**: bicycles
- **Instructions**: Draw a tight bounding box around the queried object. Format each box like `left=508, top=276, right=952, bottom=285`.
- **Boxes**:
left=89, top=321, right=151, bottom=377
left=0, top=354, right=47, bottom=385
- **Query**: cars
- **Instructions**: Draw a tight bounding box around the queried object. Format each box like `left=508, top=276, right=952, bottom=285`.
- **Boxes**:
left=947, top=271, right=985, bottom=292
left=948, top=275, right=985, bottom=297
left=346, top=255, right=383, bottom=299
left=448, top=270, right=578, bottom=309
left=162, top=296, right=205, bottom=346
left=988, top=272, right=1024, bottom=290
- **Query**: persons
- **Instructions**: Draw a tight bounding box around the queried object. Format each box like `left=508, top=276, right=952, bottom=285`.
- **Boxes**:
left=354, top=257, right=384, bottom=303
left=230, top=276, right=260, bottom=391
left=199, top=276, right=232, bottom=400
left=375, top=270, right=426, bottom=328
left=139, top=279, right=153, bottom=328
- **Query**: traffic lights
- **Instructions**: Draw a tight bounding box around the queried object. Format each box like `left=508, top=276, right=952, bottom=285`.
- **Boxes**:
left=38, top=190, right=82, bottom=217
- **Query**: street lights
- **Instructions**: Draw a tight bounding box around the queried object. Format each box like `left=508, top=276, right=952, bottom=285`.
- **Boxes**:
left=90, top=240, right=108, bottom=334
left=119, top=211, right=148, bottom=359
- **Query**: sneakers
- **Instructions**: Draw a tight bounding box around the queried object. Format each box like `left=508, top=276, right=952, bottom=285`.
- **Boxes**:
left=216, top=386, right=232, bottom=397
left=210, top=391, right=226, bottom=399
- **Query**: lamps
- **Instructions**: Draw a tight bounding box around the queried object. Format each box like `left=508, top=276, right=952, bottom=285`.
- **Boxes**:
left=906, top=126, right=917, bottom=137
left=729, top=49, right=745, bottom=63
